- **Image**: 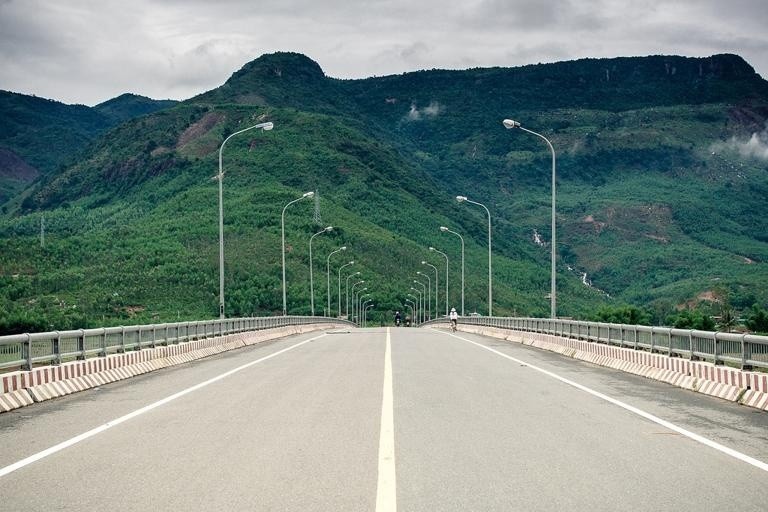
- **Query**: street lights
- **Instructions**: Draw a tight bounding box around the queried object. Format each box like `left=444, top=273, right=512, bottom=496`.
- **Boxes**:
left=502, top=118, right=556, bottom=320
left=281, top=191, right=315, bottom=316
left=310, top=227, right=334, bottom=317
left=339, top=261, right=374, bottom=328
left=439, top=226, right=465, bottom=316
left=217, top=122, right=273, bottom=318
left=327, top=246, right=347, bottom=317
left=428, top=246, right=449, bottom=316
left=456, top=195, right=492, bottom=316
left=403, top=260, right=438, bottom=326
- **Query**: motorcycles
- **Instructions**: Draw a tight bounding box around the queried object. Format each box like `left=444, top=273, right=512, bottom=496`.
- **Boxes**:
left=395, top=318, right=401, bottom=326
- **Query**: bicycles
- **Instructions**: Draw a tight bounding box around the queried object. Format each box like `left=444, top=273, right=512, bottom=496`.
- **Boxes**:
left=449, top=320, right=457, bottom=334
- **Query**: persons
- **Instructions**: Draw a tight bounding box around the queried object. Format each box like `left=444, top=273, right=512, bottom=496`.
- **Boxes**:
left=395, top=311, right=401, bottom=326
left=448, top=307, right=458, bottom=333
